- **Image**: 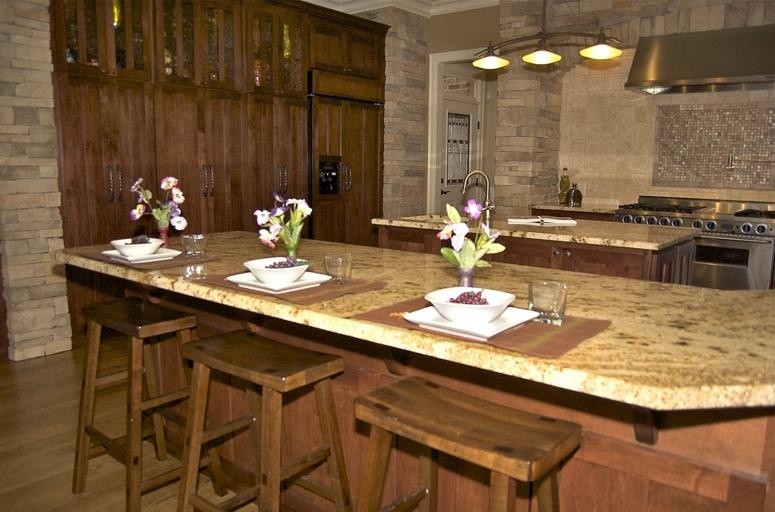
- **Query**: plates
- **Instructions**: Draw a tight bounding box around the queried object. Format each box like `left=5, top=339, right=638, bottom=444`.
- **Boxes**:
left=402, top=305, right=541, bottom=343
left=101, top=246, right=183, bottom=264
left=226, top=271, right=333, bottom=296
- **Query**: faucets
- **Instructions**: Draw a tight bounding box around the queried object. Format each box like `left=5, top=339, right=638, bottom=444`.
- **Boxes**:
left=461, top=170, right=495, bottom=219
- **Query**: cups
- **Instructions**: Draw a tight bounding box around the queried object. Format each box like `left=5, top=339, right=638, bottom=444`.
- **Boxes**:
left=323, top=252, right=354, bottom=285
left=528, top=280, right=569, bottom=326
left=181, top=231, right=207, bottom=260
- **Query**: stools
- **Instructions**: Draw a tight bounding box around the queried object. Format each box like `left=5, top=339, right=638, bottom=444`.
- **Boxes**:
left=176, top=328, right=354, bottom=512
left=353, top=376, right=583, bottom=512
left=73, top=295, right=228, bottom=512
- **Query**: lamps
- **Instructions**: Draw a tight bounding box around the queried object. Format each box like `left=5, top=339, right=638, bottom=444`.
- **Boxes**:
left=472, top=1, right=622, bottom=72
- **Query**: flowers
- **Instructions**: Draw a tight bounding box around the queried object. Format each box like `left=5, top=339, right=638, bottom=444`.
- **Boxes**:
left=254, top=192, right=313, bottom=250
left=129, top=174, right=187, bottom=230
left=437, top=200, right=506, bottom=272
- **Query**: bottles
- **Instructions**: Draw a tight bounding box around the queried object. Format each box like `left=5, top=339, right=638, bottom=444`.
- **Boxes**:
left=560, top=168, right=570, bottom=204
left=567, top=183, right=582, bottom=206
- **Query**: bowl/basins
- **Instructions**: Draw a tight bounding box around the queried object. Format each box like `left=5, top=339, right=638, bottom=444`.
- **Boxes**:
left=242, top=256, right=311, bottom=285
left=424, top=286, right=516, bottom=327
left=110, top=237, right=165, bottom=258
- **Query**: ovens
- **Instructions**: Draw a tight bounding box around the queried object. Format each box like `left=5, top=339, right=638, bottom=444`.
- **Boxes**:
left=694, top=231, right=774, bottom=290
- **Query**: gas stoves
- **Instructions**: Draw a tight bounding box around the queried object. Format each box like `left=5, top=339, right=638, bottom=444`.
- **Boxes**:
left=614, top=195, right=775, bottom=236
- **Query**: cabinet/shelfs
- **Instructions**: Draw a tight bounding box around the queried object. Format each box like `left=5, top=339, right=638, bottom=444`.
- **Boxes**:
left=371, top=214, right=701, bottom=287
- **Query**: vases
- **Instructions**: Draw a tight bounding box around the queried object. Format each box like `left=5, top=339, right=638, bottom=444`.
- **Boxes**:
left=286, top=246, right=298, bottom=259
left=458, top=268, right=472, bottom=287
left=158, top=227, right=171, bottom=250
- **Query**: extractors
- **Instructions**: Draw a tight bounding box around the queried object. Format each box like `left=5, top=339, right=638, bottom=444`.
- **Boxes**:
left=624, top=24, right=775, bottom=95
